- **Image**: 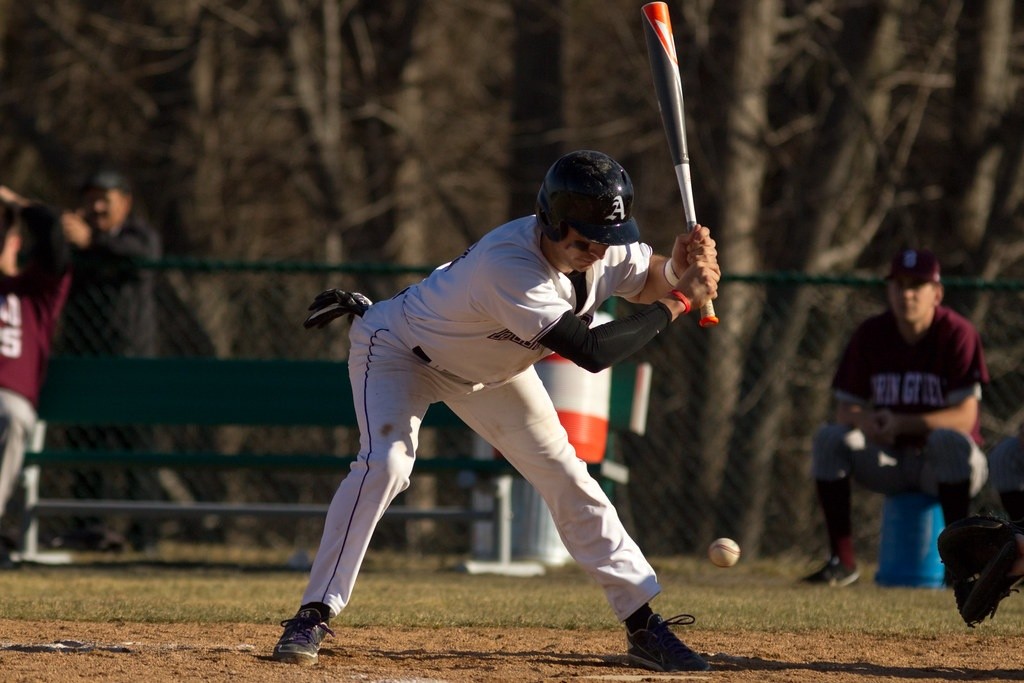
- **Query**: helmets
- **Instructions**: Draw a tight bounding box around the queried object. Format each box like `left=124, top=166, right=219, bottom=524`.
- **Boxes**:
left=536, top=150, right=640, bottom=246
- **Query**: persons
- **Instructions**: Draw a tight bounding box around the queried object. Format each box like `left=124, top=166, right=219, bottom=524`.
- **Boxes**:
left=274, top=150, right=722, bottom=672
left=799, top=250, right=989, bottom=589
left=938, top=420, right=1024, bottom=624
left=0, top=172, right=225, bottom=551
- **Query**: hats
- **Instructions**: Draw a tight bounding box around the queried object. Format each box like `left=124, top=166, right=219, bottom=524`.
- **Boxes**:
left=886, top=249, right=940, bottom=283
left=80, top=170, right=129, bottom=194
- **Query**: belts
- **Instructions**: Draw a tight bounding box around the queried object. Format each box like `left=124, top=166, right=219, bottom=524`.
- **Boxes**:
left=412, top=345, right=432, bottom=363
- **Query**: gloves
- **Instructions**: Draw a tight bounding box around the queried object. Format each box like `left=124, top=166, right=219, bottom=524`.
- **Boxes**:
left=303, top=289, right=373, bottom=329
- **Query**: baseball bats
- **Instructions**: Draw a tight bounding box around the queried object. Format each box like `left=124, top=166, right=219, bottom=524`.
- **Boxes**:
left=640, top=1, right=719, bottom=328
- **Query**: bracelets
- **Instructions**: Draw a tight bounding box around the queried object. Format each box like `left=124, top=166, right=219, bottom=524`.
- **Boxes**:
left=665, top=258, right=680, bottom=286
left=667, top=289, right=691, bottom=315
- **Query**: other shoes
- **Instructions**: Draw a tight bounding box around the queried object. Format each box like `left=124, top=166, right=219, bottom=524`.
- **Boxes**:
left=806, top=554, right=859, bottom=587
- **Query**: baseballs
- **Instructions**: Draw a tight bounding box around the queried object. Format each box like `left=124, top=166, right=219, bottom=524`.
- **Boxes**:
left=707, top=537, right=741, bottom=568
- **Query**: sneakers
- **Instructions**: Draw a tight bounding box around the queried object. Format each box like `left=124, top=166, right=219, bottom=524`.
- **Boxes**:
left=625, top=613, right=711, bottom=672
left=272, top=602, right=336, bottom=666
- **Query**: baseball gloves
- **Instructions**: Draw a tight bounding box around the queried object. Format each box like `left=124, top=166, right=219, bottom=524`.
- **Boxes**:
left=937, top=516, right=1024, bottom=624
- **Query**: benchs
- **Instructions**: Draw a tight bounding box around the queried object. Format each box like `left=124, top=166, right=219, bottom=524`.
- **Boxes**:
left=0, top=251, right=530, bottom=582
